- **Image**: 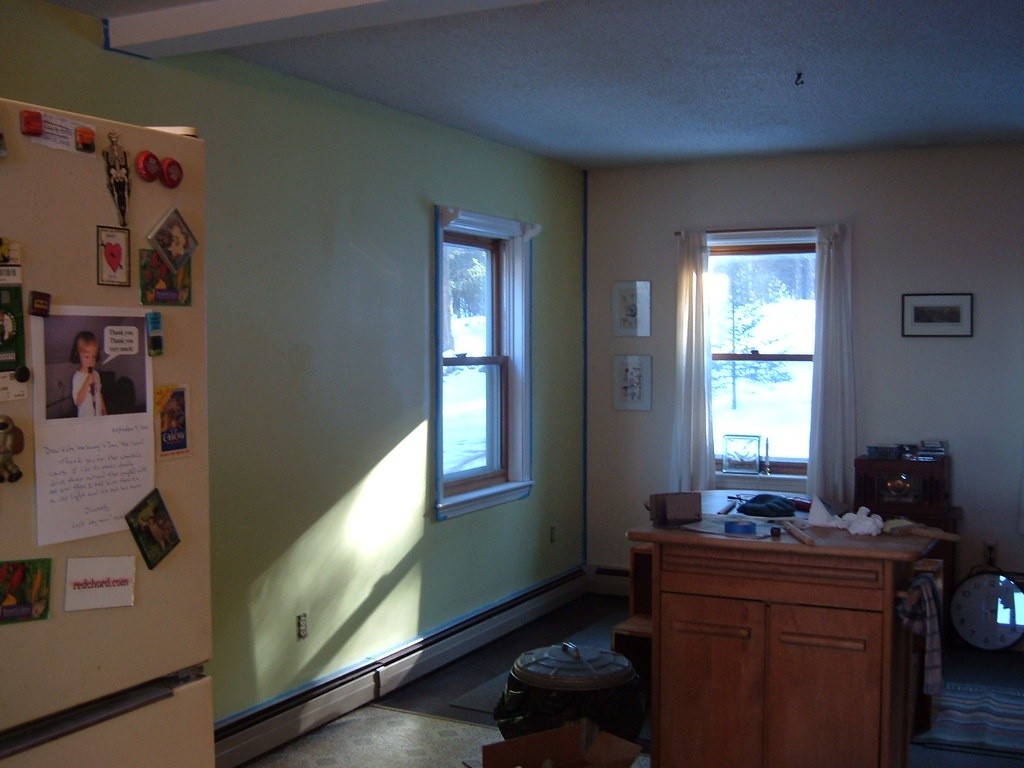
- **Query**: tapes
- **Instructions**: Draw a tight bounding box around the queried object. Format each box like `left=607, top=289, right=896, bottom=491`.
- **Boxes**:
left=724, top=520, right=756, bottom=534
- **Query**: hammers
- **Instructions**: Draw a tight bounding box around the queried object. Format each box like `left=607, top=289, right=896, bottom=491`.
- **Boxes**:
left=889, top=523, right=961, bottom=542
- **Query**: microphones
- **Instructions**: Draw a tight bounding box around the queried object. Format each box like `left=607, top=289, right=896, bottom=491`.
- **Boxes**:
left=88, top=366, right=96, bottom=408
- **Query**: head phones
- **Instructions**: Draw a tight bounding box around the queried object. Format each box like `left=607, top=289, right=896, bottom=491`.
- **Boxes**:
left=70, top=335, right=101, bottom=364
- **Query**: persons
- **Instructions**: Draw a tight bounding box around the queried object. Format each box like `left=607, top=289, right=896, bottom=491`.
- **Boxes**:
left=70, top=332, right=107, bottom=417
left=160, top=221, right=190, bottom=266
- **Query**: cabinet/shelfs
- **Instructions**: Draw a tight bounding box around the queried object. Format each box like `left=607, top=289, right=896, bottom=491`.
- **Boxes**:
left=623, top=507, right=963, bottom=768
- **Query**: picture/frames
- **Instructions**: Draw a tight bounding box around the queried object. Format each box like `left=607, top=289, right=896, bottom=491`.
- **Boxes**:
left=612, top=355, right=651, bottom=411
left=612, top=281, right=651, bottom=336
left=153, top=383, right=191, bottom=462
left=149, top=207, right=199, bottom=274
left=124, top=488, right=180, bottom=569
left=902, top=293, right=974, bottom=336
left=95, top=226, right=131, bottom=287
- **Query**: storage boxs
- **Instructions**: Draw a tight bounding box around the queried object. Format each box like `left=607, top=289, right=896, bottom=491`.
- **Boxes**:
left=649, top=492, right=703, bottom=526
left=462, top=726, right=644, bottom=768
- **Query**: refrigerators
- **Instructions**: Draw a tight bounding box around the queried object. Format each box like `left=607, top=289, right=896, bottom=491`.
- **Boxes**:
left=0, top=98, right=215, bottom=768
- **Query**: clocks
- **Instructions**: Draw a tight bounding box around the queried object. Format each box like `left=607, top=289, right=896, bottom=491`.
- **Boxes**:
left=945, top=563, right=1024, bottom=657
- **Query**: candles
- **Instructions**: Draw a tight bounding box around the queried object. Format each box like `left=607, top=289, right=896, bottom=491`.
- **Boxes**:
left=764, top=438, right=769, bottom=466
